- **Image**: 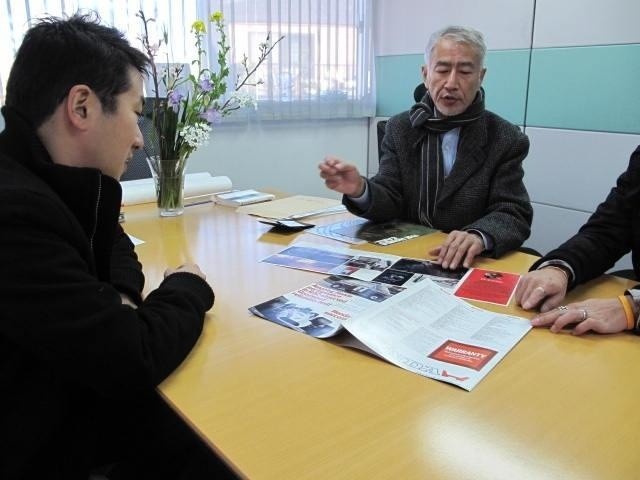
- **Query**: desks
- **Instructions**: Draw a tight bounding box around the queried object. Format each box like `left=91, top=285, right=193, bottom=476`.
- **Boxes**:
left=114, top=188, right=637, bottom=478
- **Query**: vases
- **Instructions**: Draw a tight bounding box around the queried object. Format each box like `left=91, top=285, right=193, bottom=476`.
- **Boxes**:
left=148, top=151, right=189, bottom=217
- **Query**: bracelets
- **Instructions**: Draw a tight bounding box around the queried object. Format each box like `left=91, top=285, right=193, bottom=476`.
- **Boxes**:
left=619, top=295, right=635, bottom=332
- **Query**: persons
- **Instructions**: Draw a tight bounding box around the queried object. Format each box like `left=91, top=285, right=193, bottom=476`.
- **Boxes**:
left=319, top=25, right=532, bottom=269
left=515, top=145, right=640, bottom=336
left=0, top=13, right=215, bottom=476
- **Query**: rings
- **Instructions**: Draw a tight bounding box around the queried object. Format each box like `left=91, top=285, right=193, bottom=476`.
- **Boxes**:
left=582, top=308, right=589, bottom=321
left=558, top=306, right=568, bottom=312
left=537, top=286, right=546, bottom=293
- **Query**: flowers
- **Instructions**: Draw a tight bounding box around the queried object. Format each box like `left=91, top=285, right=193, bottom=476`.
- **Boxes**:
left=120, top=8, right=285, bottom=206
